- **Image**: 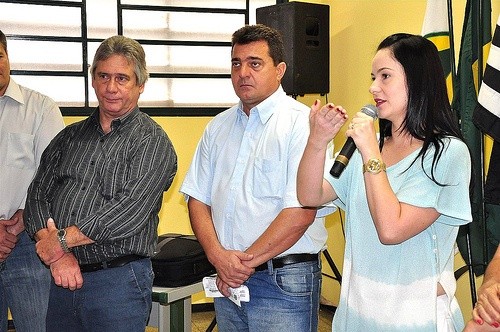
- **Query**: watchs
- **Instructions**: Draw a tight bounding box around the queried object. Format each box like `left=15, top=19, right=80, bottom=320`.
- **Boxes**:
left=363, top=159, right=386, bottom=175
left=56, top=229, right=72, bottom=253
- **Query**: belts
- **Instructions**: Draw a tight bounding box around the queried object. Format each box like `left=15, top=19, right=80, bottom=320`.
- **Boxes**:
left=79, top=253, right=149, bottom=273
left=255, top=253, right=318, bottom=272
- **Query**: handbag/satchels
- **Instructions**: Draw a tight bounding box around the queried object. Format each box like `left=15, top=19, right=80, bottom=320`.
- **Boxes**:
left=151, top=234, right=217, bottom=288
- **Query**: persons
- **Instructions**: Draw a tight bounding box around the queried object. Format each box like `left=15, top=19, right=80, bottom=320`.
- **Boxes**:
left=297, top=33, right=472, bottom=332
left=23, top=36, right=177, bottom=332
left=179, top=23, right=334, bottom=332
left=0, top=30, right=67, bottom=332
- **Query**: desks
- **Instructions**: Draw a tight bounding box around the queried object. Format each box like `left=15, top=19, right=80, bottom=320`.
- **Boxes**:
left=152, top=281, right=204, bottom=332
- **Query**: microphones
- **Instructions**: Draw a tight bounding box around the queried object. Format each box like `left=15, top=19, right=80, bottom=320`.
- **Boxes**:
left=330, top=104, right=378, bottom=178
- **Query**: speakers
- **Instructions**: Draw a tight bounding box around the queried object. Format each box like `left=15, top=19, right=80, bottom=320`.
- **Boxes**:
left=255, top=1, right=330, bottom=94
